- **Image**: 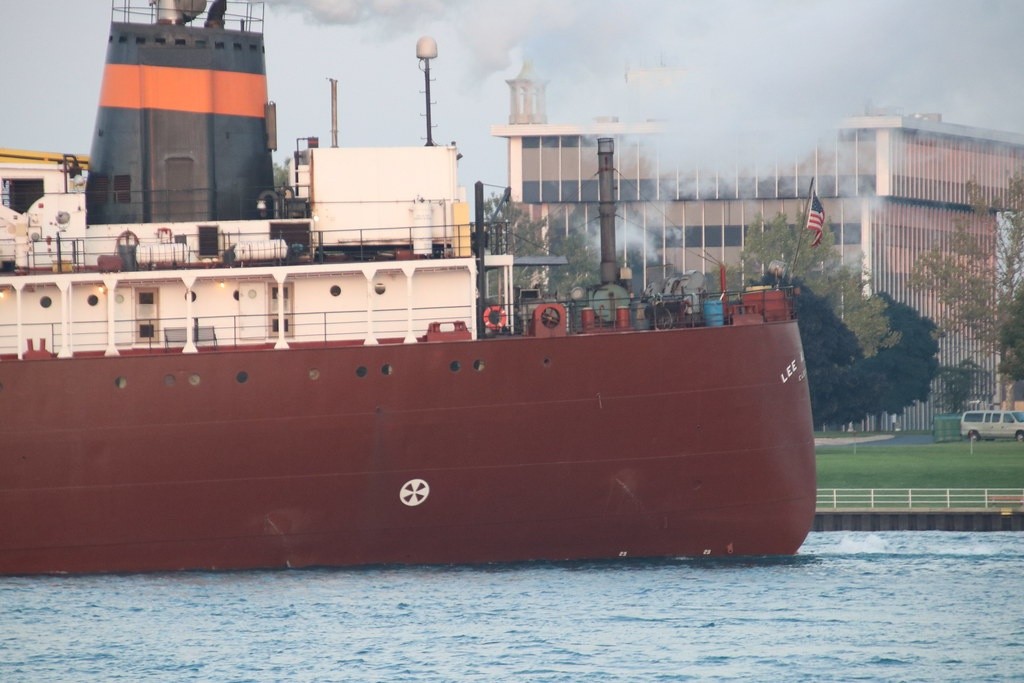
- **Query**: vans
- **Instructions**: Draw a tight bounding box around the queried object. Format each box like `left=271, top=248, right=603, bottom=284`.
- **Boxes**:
left=959, top=409, right=1024, bottom=444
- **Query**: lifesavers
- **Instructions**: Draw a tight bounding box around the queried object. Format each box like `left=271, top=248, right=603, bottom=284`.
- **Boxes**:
left=483, top=305, right=507, bottom=330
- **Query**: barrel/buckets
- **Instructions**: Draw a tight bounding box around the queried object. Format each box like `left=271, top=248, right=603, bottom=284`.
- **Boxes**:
left=631, top=301, right=650, bottom=330
left=616, top=306, right=630, bottom=331
left=118, top=245, right=137, bottom=271
left=704, top=299, right=723, bottom=326
left=583, top=308, right=595, bottom=332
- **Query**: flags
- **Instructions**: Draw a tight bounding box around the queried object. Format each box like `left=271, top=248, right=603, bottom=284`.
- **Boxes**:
left=807, top=189, right=825, bottom=248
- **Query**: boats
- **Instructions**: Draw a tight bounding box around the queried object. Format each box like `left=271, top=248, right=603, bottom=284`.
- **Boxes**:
left=0, top=1, right=818, bottom=575
